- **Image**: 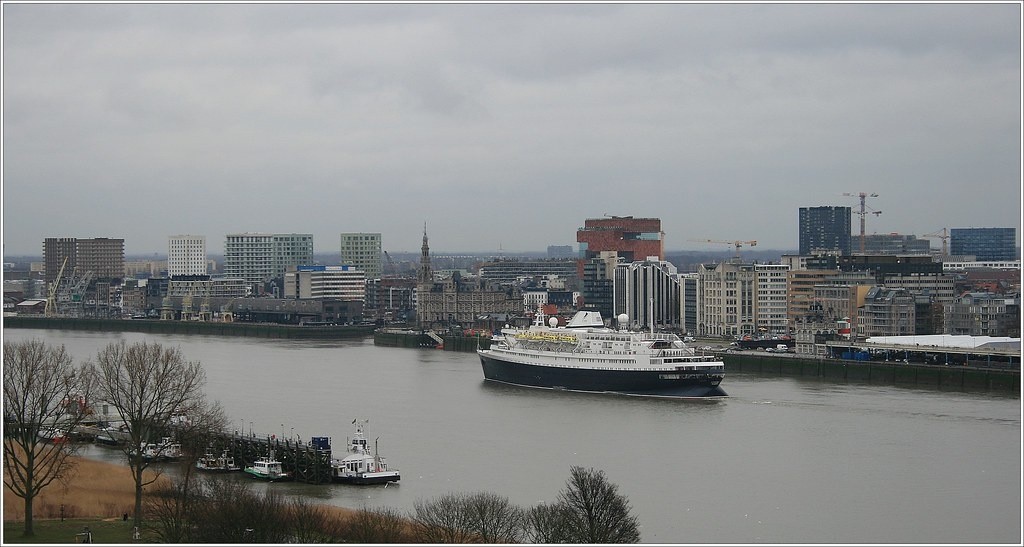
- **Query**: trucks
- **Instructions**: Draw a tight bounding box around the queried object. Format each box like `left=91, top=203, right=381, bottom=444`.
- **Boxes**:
left=777, top=344, right=789, bottom=350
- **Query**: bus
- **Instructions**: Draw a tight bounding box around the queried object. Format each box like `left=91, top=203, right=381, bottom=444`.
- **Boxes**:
left=302, top=322, right=335, bottom=327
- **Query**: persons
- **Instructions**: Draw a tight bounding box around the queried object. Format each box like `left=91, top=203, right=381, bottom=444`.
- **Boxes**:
left=123, top=512, right=128, bottom=522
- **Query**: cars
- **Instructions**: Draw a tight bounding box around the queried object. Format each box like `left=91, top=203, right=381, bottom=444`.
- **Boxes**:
left=756, top=347, right=763, bottom=350
left=132, top=314, right=144, bottom=319
left=678, top=336, right=743, bottom=352
left=766, top=348, right=774, bottom=352
left=344, top=320, right=406, bottom=326
left=885, top=358, right=967, bottom=366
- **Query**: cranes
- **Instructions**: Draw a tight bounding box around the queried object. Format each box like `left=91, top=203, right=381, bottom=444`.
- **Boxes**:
left=685, top=239, right=757, bottom=258
left=923, top=227, right=951, bottom=255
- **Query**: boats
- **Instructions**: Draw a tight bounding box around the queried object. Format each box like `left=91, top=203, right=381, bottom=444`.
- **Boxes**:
left=60, top=390, right=95, bottom=415
left=475, top=310, right=726, bottom=399
left=329, top=419, right=401, bottom=485
left=13, top=421, right=294, bottom=482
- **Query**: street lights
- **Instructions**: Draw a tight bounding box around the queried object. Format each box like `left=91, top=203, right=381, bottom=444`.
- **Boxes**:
left=942, top=336, right=944, bottom=348
left=280, top=424, right=285, bottom=444
left=291, top=427, right=294, bottom=444
left=973, top=337, right=975, bottom=351
left=1005, top=339, right=1007, bottom=352
left=249, top=421, right=253, bottom=442
left=241, top=418, right=244, bottom=437
